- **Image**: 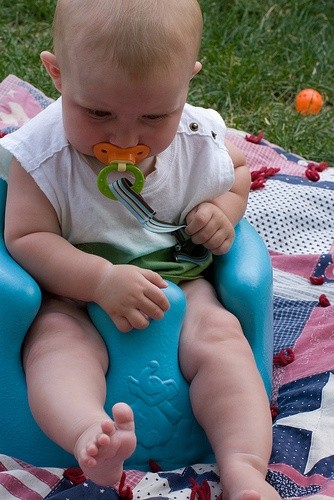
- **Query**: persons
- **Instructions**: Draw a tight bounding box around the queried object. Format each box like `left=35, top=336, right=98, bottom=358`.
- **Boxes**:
left=0, top=0, right=281, bottom=500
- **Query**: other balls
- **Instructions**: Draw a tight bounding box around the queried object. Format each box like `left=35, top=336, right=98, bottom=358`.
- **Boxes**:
left=296, top=88, right=323, bottom=115
